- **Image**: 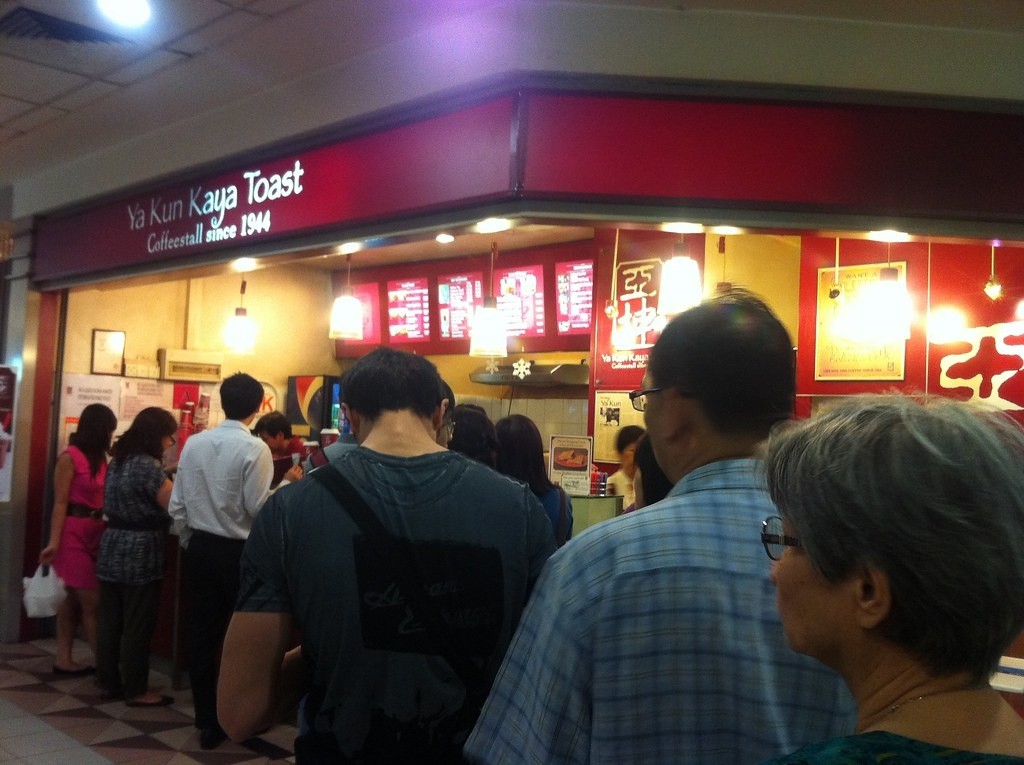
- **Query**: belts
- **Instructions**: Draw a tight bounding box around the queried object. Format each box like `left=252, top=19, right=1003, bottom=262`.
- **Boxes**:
left=65, top=502, right=101, bottom=519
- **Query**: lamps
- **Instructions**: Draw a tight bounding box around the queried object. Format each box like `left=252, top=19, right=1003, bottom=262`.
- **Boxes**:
left=469, top=236, right=508, bottom=359
left=983, top=245, right=1002, bottom=301
left=657, top=232, right=703, bottom=317
left=870, top=241, right=911, bottom=342
left=222, top=271, right=257, bottom=348
left=328, top=253, right=364, bottom=341
left=603, top=227, right=619, bottom=319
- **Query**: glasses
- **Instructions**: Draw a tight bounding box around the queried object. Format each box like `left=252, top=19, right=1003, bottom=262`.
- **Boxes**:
left=441, top=421, right=455, bottom=441
left=630, top=387, right=701, bottom=412
left=760, top=517, right=801, bottom=561
left=166, top=433, right=176, bottom=448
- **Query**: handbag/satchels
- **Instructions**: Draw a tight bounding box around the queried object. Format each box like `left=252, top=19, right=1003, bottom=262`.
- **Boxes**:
left=23, top=562, right=69, bottom=618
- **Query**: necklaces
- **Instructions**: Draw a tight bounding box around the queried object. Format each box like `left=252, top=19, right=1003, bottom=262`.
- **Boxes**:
left=890, top=681, right=993, bottom=711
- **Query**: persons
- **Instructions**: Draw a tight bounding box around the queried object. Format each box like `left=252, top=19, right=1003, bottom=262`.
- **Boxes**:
left=168, top=374, right=305, bottom=751
left=757, top=389, right=1024, bottom=765
left=624, top=432, right=675, bottom=517
left=39, top=405, right=117, bottom=676
left=606, top=424, right=647, bottom=512
left=215, top=346, right=558, bottom=764
left=249, top=412, right=306, bottom=462
left=464, top=287, right=859, bottom=765
left=102, top=407, right=178, bottom=708
left=435, top=380, right=573, bottom=548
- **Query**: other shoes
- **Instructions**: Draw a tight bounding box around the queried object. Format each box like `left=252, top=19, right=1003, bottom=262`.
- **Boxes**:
left=200, top=725, right=227, bottom=750
left=54, top=664, right=95, bottom=676
left=128, top=693, right=174, bottom=707
left=101, top=686, right=125, bottom=699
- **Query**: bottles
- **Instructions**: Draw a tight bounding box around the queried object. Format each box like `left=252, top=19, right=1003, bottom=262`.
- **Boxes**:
left=590, top=472, right=607, bottom=496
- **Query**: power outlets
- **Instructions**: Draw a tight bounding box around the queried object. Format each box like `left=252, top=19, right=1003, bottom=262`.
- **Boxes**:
left=125, top=363, right=138, bottom=377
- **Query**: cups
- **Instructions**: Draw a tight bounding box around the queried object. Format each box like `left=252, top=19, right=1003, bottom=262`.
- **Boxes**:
left=176, top=411, right=191, bottom=461
left=320, top=428, right=339, bottom=448
left=303, top=440, right=319, bottom=461
left=192, top=392, right=211, bottom=434
left=183, top=402, right=195, bottom=435
left=0, top=433, right=12, bottom=469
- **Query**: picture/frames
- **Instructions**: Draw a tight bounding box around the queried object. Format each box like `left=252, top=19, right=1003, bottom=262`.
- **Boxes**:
left=90, top=328, right=126, bottom=377
